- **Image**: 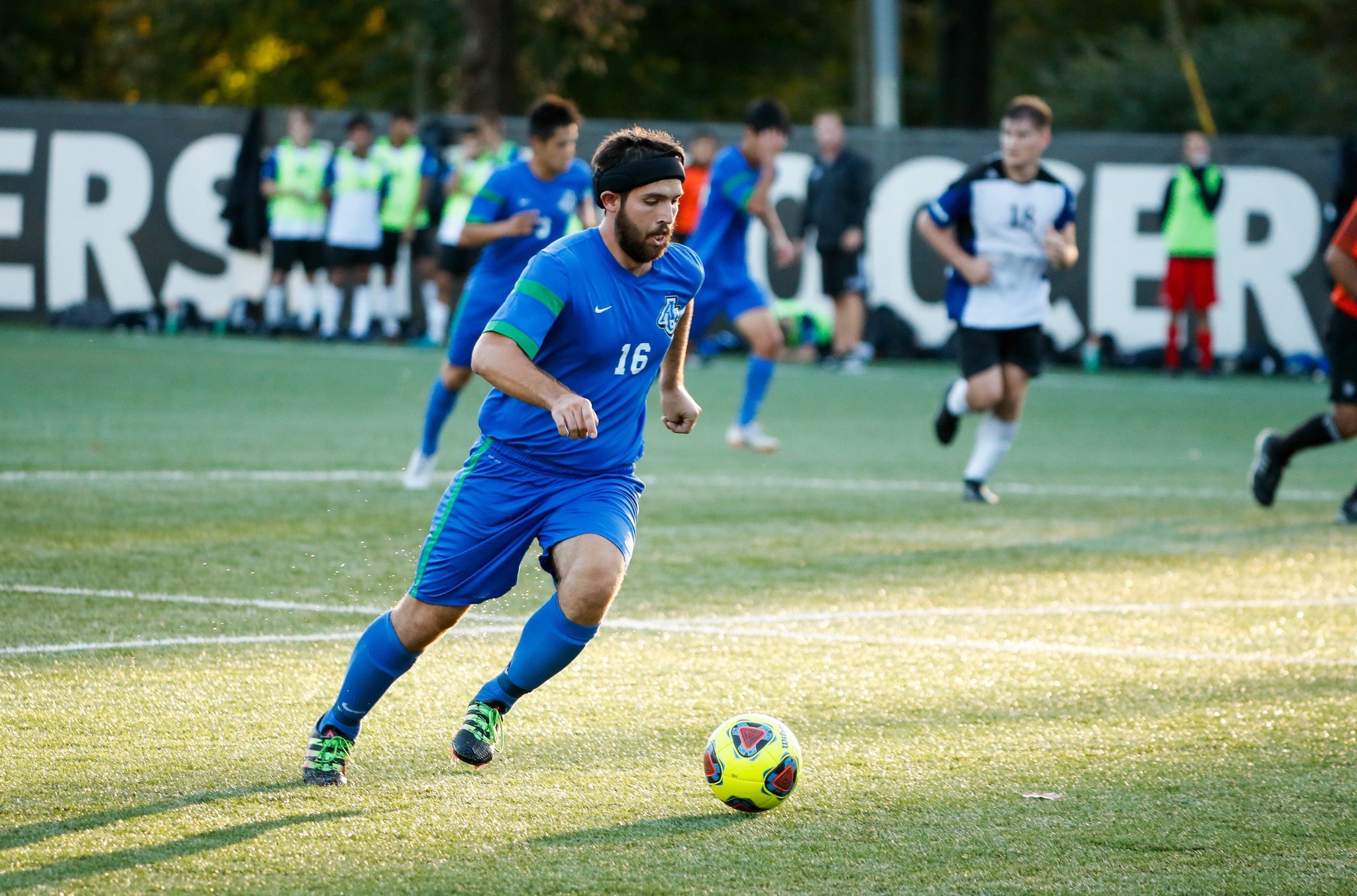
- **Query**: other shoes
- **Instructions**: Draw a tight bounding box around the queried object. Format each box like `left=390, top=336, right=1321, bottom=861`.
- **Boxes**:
left=727, top=423, right=778, bottom=452
left=961, top=478, right=1001, bottom=506
left=404, top=448, right=441, bottom=490
left=936, top=382, right=960, bottom=444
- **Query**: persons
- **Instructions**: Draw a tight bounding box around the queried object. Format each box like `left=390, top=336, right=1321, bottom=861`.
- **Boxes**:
left=1251, top=198, right=1357, bottom=523
left=258, top=109, right=519, bottom=345
left=687, top=100, right=796, bottom=452
left=1159, top=129, right=1224, bottom=374
left=796, top=109, right=870, bottom=369
left=675, top=133, right=718, bottom=242
left=305, top=125, right=706, bottom=785
left=405, top=96, right=597, bottom=493
left=917, top=97, right=1079, bottom=503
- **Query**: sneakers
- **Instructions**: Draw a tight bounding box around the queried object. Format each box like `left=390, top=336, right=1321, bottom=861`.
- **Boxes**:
left=451, top=699, right=508, bottom=770
left=1337, top=496, right=1357, bottom=525
left=304, top=711, right=355, bottom=787
left=1247, top=428, right=1288, bottom=507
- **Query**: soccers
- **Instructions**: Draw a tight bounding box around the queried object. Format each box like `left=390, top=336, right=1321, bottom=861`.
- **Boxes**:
left=702, top=712, right=803, bottom=813
